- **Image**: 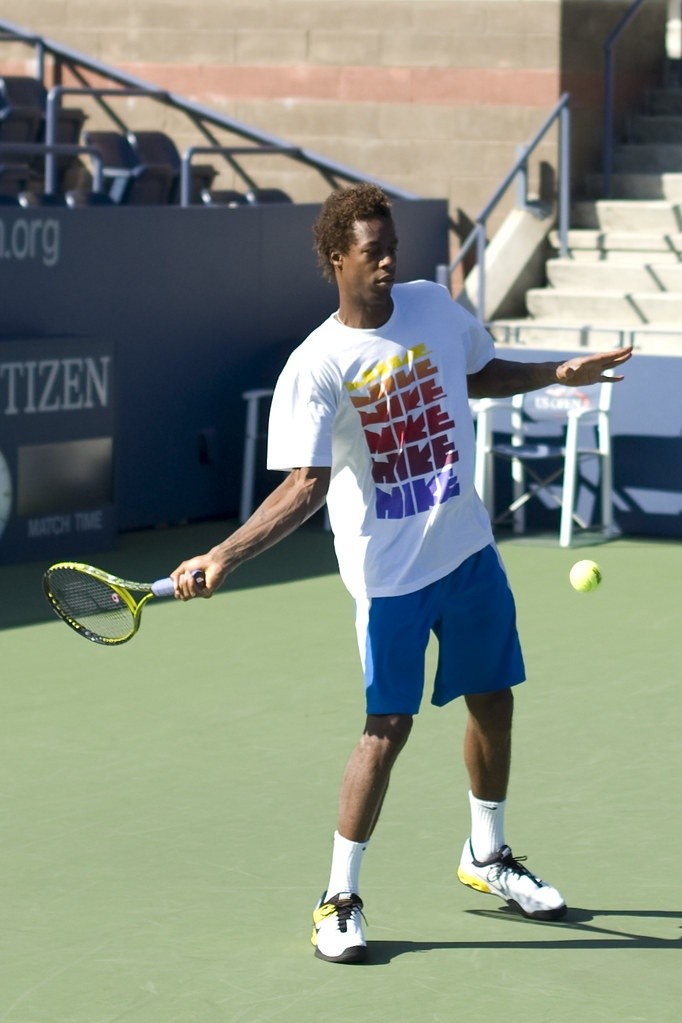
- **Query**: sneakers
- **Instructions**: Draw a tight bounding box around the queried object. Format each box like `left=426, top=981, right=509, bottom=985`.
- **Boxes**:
left=456, top=839, right=569, bottom=920
left=310, top=889, right=370, bottom=962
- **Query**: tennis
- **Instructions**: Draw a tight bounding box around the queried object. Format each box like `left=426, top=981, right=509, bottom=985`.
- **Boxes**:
left=570, top=560, right=602, bottom=592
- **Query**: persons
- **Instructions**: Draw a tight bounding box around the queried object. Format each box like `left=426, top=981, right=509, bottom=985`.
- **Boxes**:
left=170, top=180, right=635, bottom=966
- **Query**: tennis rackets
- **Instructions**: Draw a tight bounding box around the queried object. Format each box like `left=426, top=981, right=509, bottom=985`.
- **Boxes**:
left=43, top=561, right=205, bottom=645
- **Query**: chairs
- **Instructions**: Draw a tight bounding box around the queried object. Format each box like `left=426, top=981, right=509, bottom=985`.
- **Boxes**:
left=473, top=369, right=618, bottom=551
left=0, top=75, right=298, bottom=208
left=240, top=388, right=331, bottom=531
